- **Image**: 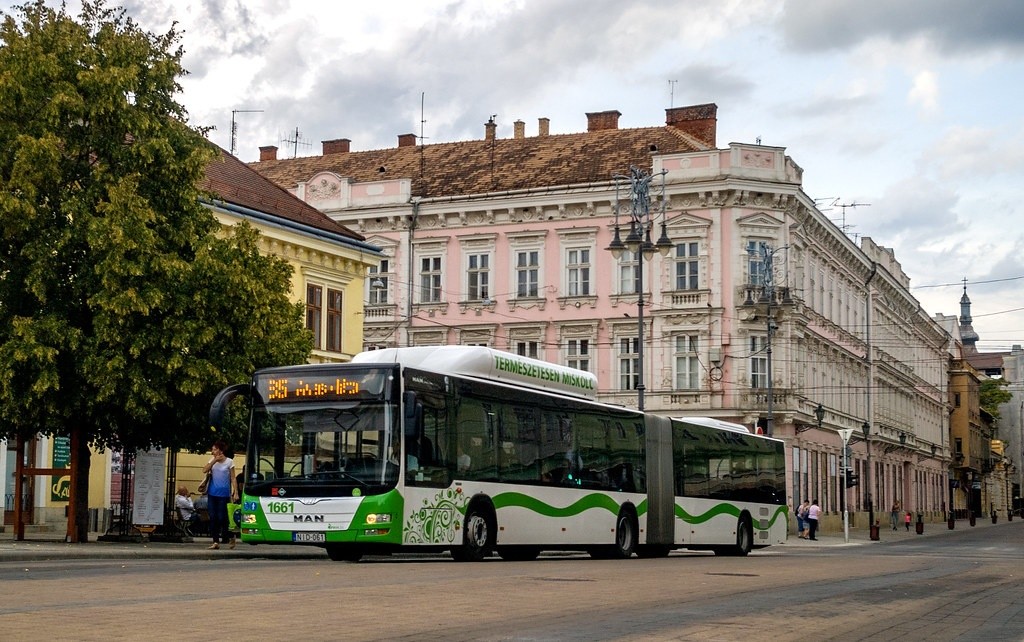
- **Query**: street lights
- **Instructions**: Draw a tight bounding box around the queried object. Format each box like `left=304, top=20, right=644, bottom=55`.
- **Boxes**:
left=740, top=243, right=804, bottom=437
left=604, top=165, right=677, bottom=415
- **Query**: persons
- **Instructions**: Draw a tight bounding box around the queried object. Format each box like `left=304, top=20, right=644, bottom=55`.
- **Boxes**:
left=193, top=492, right=210, bottom=511
left=174, top=486, right=195, bottom=536
left=892, top=500, right=901, bottom=531
left=201, top=440, right=240, bottom=551
left=796, top=500, right=823, bottom=542
left=560, top=450, right=583, bottom=488
left=457, top=445, right=471, bottom=475
left=905, top=513, right=912, bottom=532
left=383, top=439, right=420, bottom=473
left=235, top=464, right=245, bottom=502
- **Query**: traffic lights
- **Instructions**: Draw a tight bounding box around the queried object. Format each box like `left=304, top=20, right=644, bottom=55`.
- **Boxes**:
left=846, top=469, right=858, bottom=488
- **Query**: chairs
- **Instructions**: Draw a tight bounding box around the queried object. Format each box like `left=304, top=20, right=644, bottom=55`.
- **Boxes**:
left=173, top=506, right=210, bottom=536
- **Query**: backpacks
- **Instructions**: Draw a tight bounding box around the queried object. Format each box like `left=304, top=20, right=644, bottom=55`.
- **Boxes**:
left=795, top=506, right=800, bottom=517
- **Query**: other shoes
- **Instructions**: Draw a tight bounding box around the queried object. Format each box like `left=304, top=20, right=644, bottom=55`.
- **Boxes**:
left=229, top=538, right=235, bottom=549
left=810, top=538, right=818, bottom=542
left=208, top=544, right=220, bottom=550
left=804, top=537, right=810, bottom=540
left=798, top=535, right=805, bottom=538
left=187, top=527, right=195, bottom=537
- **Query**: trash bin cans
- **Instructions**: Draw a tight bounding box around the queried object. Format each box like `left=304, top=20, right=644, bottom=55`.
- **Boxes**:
left=915, top=514, right=923, bottom=534
left=970, top=511, right=976, bottom=526
left=871, top=520, right=880, bottom=540
left=948, top=512, right=955, bottom=529
left=992, top=511, right=998, bottom=524
left=1008, top=509, right=1013, bottom=521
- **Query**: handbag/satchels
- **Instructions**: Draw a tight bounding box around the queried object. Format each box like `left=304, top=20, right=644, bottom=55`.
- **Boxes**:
left=198, top=470, right=212, bottom=494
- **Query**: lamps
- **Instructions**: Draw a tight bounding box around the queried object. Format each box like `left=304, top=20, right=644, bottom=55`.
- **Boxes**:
left=372, top=278, right=384, bottom=288
left=885, top=432, right=907, bottom=454
left=846, top=422, right=871, bottom=445
left=482, top=297, right=492, bottom=305
left=919, top=442, right=937, bottom=461
left=795, top=404, right=825, bottom=435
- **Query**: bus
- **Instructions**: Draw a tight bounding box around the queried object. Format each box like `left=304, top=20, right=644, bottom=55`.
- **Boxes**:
left=209, top=346, right=790, bottom=563
